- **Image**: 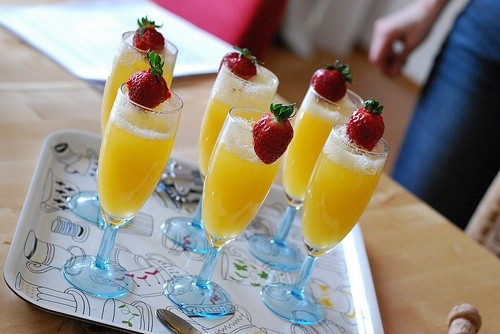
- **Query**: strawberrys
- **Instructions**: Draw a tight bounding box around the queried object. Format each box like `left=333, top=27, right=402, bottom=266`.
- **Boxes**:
left=218, top=47, right=260, bottom=80
left=126, top=48, right=171, bottom=110
left=252, top=103, right=297, bottom=165
left=310, top=59, right=353, bottom=104
left=346, top=98, right=385, bottom=152
left=133, top=16, right=165, bottom=61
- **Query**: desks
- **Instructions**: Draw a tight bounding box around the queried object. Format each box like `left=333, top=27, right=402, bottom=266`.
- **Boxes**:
left=1, top=1, right=500, bottom=333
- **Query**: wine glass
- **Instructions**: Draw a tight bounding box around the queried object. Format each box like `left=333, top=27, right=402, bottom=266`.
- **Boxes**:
left=163, top=106, right=287, bottom=317
left=62, top=84, right=183, bottom=299
left=260, top=124, right=390, bottom=325
left=68, top=30, right=179, bottom=227
left=160, top=54, right=280, bottom=254
left=249, top=86, right=363, bottom=270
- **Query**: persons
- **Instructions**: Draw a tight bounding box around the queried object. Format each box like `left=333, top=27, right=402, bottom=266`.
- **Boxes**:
left=367, top=0, right=500, bottom=231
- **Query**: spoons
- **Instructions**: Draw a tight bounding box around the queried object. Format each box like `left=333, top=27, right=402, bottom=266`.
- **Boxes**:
left=155, top=308, right=201, bottom=334
